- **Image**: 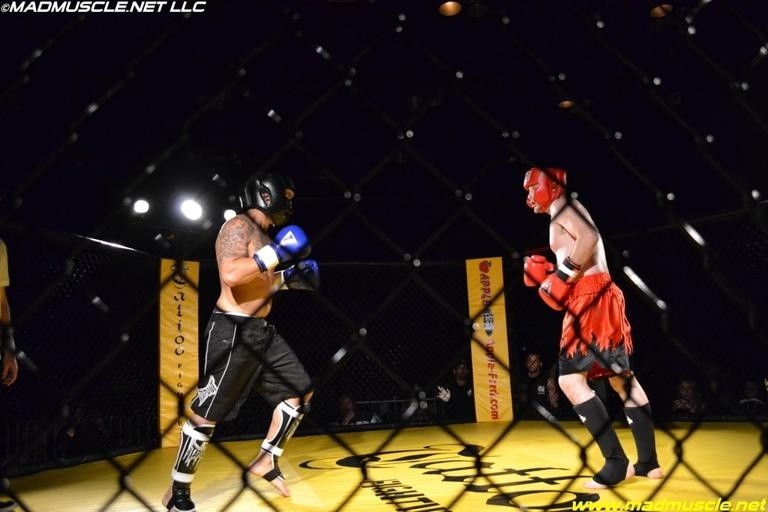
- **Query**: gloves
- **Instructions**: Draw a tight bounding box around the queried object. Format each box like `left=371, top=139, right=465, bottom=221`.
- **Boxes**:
left=538, top=256, right=585, bottom=312
left=523, top=254, right=558, bottom=287
left=273, top=260, right=321, bottom=293
left=253, top=224, right=312, bottom=274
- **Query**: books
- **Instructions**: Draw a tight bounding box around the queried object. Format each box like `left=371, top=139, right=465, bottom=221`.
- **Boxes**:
left=520, top=166, right=664, bottom=490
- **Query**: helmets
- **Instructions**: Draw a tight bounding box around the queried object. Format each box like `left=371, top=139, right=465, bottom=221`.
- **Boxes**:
left=523, top=164, right=571, bottom=214
left=239, top=169, right=297, bottom=216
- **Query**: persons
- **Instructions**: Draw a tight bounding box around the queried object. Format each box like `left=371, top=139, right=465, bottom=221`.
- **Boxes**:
left=159, top=163, right=321, bottom=512
left=737, top=380, right=764, bottom=414
left=513, top=351, right=559, bottom=417
left=57, top=401, right=84, bottom=461
left=434, top=361, right=472, bottom=419
left=670, top=379, right=707, bottom=414
left=0, top=240, right=22, bottom=510
left=333, top=393, right=370, bottom=424
